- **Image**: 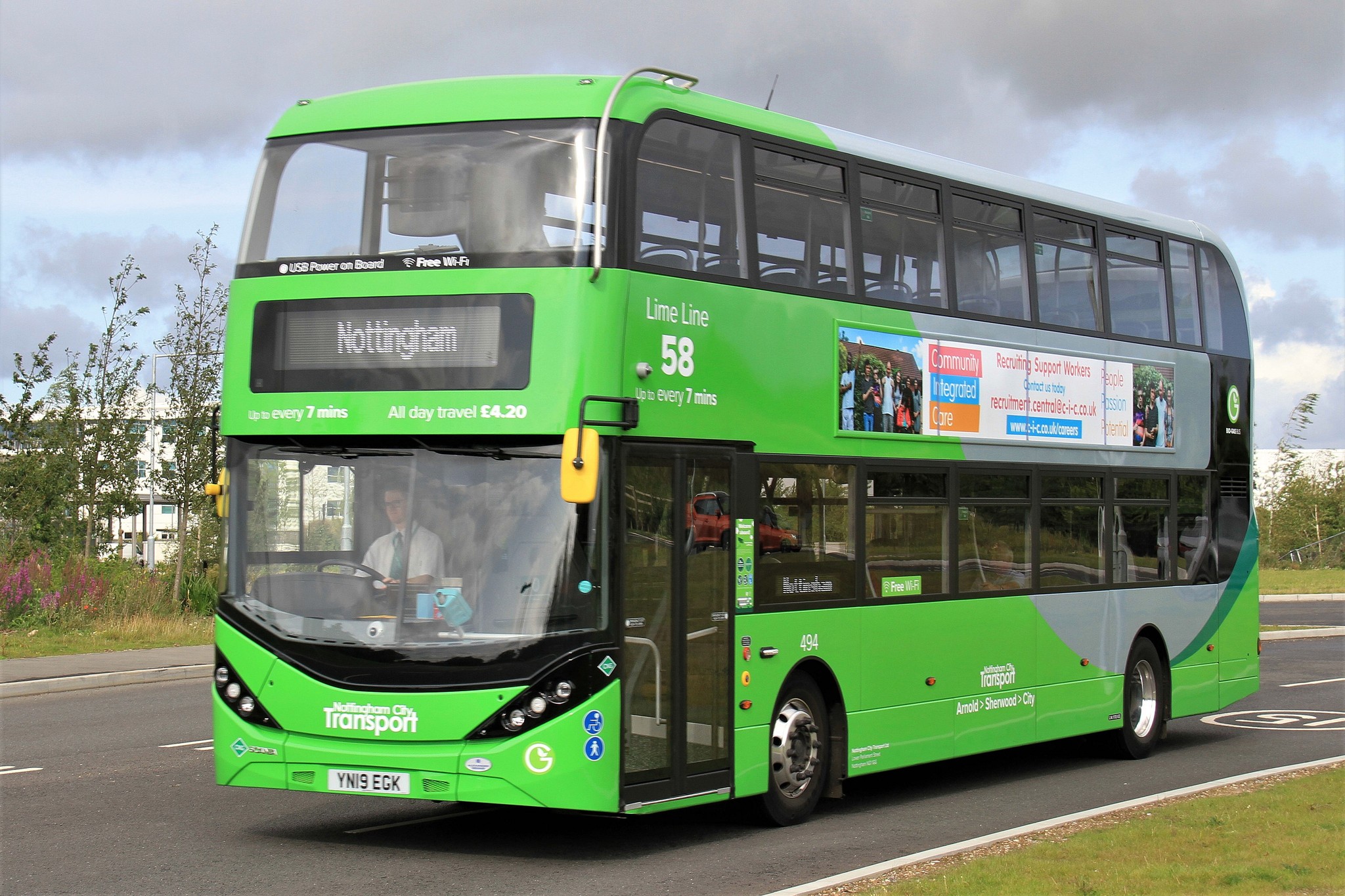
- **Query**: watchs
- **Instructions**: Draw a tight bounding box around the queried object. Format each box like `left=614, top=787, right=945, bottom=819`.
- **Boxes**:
left=998, top=585, right=1004, bottom=590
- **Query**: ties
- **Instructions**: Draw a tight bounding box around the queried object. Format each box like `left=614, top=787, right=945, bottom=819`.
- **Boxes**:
left=389, top=533, right=404, bottom=580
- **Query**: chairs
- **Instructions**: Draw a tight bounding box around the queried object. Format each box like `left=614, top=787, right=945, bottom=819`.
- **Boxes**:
left=432, top=517, right=477, bottom=609
left=636, top=244, right=1149, bottom=338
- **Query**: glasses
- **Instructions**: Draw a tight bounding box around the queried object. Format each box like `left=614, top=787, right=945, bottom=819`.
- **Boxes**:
left=381, top=499, right=402, bottom=509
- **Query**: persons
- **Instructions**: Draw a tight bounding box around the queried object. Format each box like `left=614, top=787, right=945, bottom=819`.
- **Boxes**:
left=1133, top=379, right=1174, bottom=447
left=840, top=340, right=921, bottom=434
left=350, top=487, right=444, bottom=591
left=970, top=540, right=1025, bottom=591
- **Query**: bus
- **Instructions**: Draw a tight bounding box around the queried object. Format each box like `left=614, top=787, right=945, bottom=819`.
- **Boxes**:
left=205, top=67, right=1266, bottom=831
